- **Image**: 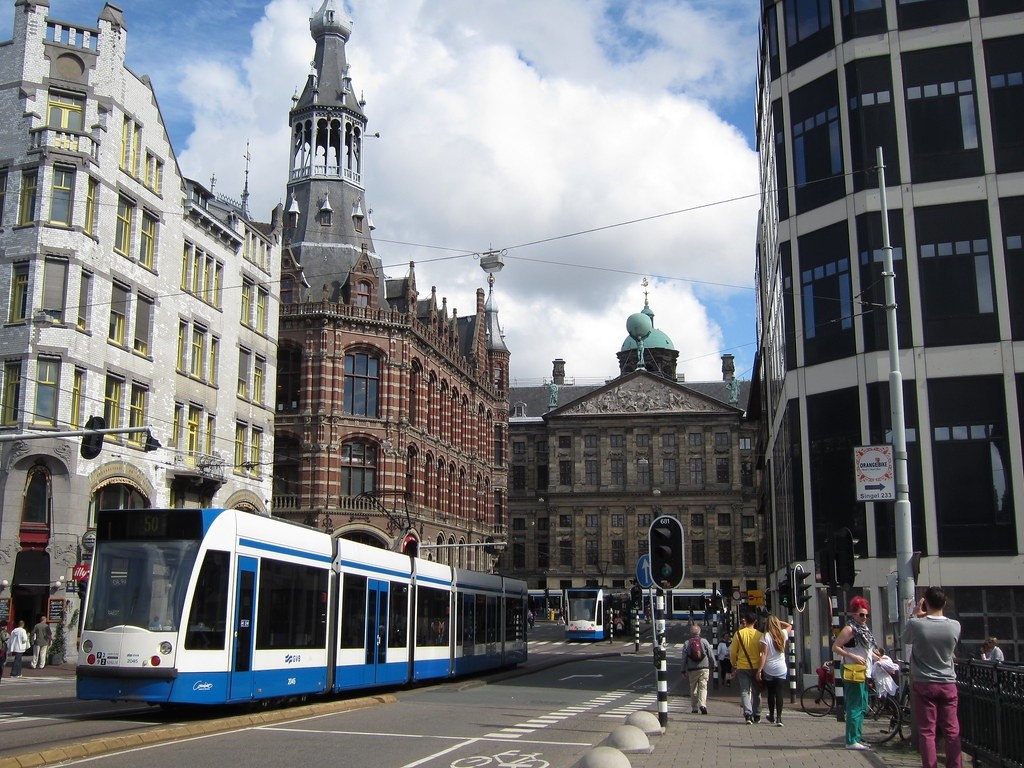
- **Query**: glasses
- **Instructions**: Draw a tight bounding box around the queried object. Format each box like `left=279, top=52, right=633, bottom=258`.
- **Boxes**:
left=856, top=613, right=870, bottom=618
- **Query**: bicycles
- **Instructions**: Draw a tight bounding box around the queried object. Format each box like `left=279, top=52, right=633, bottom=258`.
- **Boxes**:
left=800, top=648, right=913, bottom=744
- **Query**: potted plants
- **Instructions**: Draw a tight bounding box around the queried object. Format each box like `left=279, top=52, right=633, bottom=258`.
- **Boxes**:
left=48, top=645, right=63, bottom=665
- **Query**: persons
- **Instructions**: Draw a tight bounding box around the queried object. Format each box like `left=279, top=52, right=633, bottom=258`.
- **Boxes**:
left=688, top=606, right=694, bottom=626
left=0, top=620, right=9, bottom=679
left=756, top=615, right=792, bottom=726
left=832, top=598, right=880, bottom=750
left=717, top=632, right=732, bottom=687
left=729, top=612, right=764, bottom=724
left=980, top=637, right=1004, bottom=663
left=31, top=616, right=52, bottom=668
left=902, top=587, right=963, bottom=768
left=644, top=608, right=651, bottom=623
left=703, top=607, right=709, bottom=626
left=720, top=610, right=726, bottom=627
left=680, top=625, right=715, bottom=714
left=528, top=610, right=537, bottom=627
left=8, top=621, right=31, bottom=678
left=557, top=607, right=565, bottom=625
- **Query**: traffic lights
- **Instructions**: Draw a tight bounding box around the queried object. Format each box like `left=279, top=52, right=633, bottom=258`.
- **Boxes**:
left=648, top=515, right=685, bottom=590
left=544, top=589, right=549, bottom=598
left=836, top=526, right=861, bottom=589
left=793, top=564, right=812, bottom=612
left=779, top=580, right=792, bottom=608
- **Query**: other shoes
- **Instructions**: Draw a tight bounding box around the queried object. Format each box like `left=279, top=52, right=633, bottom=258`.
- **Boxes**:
left=859, top=740, right=871, bottom=747
left=746, top=715, right=753, bottom=725
left=754, top=715, right=761, bottom=723
left=776, top=719, right=784, bottom=727
left=699, top=706, right=707, bottom=714
left=845, top=742, right=870, bottom=750
left=692, top=710, right=698, bottom=713
left=766, top=714, right=775, bottom=724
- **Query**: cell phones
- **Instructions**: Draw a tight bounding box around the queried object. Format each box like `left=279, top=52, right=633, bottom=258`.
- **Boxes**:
left=922, top=602, right=926, bottom=612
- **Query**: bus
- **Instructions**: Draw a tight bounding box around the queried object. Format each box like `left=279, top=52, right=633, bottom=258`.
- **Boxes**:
left=528, top=588, right=725, bottom=622
left=74, top=489, right=528, bottom=714
left=564, top=560, right=630, bottom=640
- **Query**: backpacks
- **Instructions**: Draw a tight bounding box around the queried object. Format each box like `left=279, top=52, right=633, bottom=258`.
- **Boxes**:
left=687, top=636, right=706, bottom=663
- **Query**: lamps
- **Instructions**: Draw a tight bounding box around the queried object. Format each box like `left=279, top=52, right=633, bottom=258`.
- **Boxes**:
left=55, top=581, right=61, bottom=590
left=2, top=580, right=8, bottom=590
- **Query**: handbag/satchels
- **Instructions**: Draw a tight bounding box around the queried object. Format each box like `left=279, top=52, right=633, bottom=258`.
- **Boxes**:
left=751, top=669, right=767, bottom=693
left=843, top=664, right=866, bottom=683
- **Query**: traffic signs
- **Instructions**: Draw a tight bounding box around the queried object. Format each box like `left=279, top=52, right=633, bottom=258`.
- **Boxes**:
left=854, top=445, right=897, bottom=502
